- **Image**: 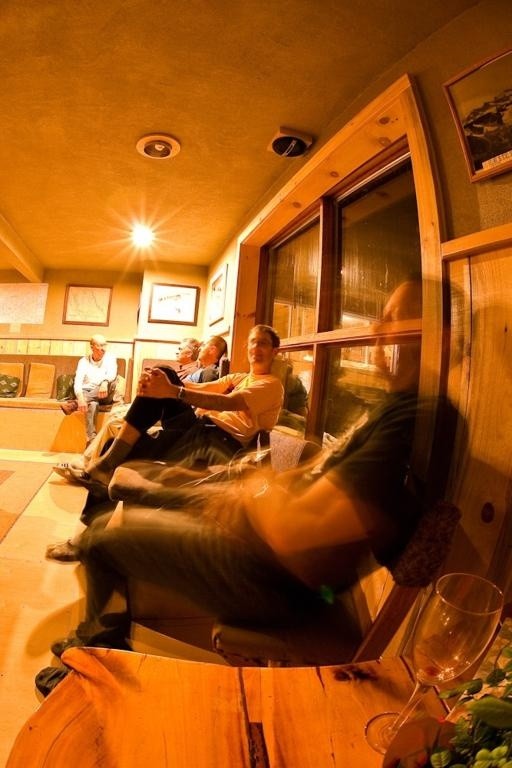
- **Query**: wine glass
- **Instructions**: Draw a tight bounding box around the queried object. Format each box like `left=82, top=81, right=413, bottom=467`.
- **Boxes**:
left=365, top=571, right=507, bottom=754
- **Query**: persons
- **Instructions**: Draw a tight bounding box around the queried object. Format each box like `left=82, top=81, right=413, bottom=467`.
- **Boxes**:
left=60, top=334, right=119, bottom=449
left=105, top=337, right=200, bottom=423
left=45, top=325, right=286, bottom=563
left=52, top=335, right=226, bottom=485
left=34, top=277, right=477, bottom=705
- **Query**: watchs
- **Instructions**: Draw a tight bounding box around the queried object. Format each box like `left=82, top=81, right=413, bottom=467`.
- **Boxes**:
left=178, top=387, right=185, bottom=403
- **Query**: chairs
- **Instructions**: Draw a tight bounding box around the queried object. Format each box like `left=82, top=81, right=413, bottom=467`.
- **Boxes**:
left=123, top=502, right=463, bottom=664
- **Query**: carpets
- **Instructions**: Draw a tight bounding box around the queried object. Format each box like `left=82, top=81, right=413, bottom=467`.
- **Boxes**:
left=1, top=458, right=59, bottom=541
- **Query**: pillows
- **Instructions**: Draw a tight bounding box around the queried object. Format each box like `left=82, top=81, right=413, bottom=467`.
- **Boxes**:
left=56, top=373, right=76, bottom=402
left=0, top=373, right=20, bottom=398
left=113, top=374, right=127, bottom=402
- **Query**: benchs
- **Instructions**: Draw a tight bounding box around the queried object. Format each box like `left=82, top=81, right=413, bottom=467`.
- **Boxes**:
left=0, top=354, right=126, bottom=454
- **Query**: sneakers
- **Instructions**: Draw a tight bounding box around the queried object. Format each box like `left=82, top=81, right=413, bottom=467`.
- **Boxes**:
left=52, top=463, right=108, bottom=489
left=61, top=400, right=78, bottom=415
left=48, top=540, right=82, bottom=560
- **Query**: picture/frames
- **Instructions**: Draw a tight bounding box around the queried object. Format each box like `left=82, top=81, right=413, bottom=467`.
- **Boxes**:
left=207, top=263, right=228, bottom=326
left=147, top=282, right=201, bottom=326
left=443, top=46, right=511, bottom=184
left=61, top=282, right=113, bottom=326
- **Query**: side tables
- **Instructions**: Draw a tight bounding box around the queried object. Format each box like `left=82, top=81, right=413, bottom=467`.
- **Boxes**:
left=5, top=645, right=504, bottom=768
